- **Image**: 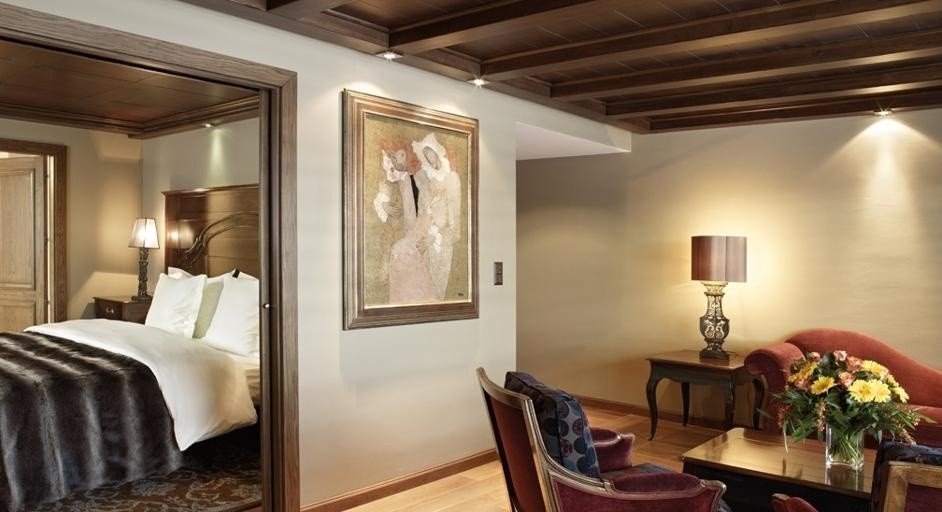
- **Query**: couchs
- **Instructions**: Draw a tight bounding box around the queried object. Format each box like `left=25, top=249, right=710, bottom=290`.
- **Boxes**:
left=744, top=327, right=942, bottom=448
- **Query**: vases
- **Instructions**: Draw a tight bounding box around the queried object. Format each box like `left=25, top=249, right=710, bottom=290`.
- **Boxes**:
left=825, top=420, right=865, bottom=473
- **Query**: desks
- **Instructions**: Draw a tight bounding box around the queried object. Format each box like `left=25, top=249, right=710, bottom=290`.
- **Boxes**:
left=644, top=349, right=766, bottom=442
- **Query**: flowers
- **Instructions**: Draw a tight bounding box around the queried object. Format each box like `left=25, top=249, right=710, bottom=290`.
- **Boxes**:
left=756, top=347, right=938, bottom=462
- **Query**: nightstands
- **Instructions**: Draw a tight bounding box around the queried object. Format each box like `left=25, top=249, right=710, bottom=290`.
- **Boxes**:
left=91, top=294, right=154, bottom=325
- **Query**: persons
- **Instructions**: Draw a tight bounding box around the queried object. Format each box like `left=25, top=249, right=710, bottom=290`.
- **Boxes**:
left=381, top=132, right=462, bottom=307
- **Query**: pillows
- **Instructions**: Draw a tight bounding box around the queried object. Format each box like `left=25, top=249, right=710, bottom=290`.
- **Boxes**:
left=166, top=263, right=237, bottom=339
left=143, top=272, right=209, bottom=340
left=503, top=371, right=602, bottom=480
left=869, top=440, right=941, bottom=510
left=197, top=273, right=261, bottom=358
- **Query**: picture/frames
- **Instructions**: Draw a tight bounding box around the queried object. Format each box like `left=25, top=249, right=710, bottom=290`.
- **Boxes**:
left=340, top=86, right=479, bottom=331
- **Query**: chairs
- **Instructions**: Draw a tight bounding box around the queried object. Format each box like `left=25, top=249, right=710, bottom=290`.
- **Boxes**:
left=474, top=364, right=727, bottom=512
left=770, top=490, right=818, bottom=512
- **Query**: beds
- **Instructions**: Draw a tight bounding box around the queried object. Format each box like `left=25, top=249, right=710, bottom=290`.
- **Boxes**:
left=0, top=182, right=261, bottom=511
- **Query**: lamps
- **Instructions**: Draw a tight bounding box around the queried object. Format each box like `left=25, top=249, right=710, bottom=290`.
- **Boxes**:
left=128, top=217, right=162, bottom=302
left=873, top=109, right=893, bottom=116
left=689, top=232, right=748, bottom=360
